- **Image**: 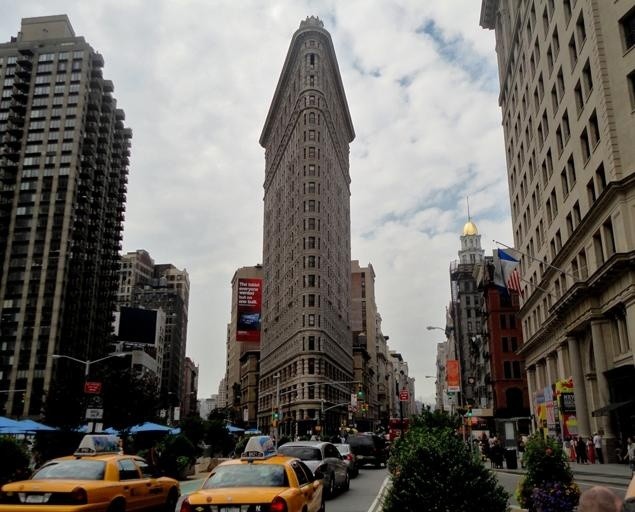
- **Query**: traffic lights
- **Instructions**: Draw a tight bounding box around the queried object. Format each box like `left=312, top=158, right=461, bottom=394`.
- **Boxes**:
left=272, top=406, right=279, bottom=426
left=357, top=385, right=363, bottom=398
left=361, top=403, right=369, bottom=413
left=466, top=411, right=472, bottom=426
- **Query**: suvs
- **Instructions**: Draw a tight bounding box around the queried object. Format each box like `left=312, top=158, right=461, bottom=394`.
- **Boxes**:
left=350, top=435, right=387, bottom=468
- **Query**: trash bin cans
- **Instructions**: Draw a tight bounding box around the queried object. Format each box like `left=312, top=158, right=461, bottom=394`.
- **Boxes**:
left=506, top=449, right=517, bottom=468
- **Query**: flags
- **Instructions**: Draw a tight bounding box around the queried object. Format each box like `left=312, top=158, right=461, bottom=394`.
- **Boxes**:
left=491, top=259, right=525, bottom=298
left=489, top=239, right=523, bottom=290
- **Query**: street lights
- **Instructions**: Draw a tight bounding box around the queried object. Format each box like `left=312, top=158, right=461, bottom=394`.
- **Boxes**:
left=51, top=353, right=126, bottom=415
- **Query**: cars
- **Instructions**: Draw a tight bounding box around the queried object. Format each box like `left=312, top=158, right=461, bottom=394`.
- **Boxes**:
left=182, top=437, right=325, bottom=512
left=277, top=441, right=359, bottom=498
left=1, top=434, right=180, bottom=511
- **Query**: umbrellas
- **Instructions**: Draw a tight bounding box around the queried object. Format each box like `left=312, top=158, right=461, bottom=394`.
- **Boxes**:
left=1, top=418, right=62, bottom=442
left=72, top=420, right=121, bottom=436
left=0, top=416, right=37, bottom=435
left=118, top=420, right=176, bottom=435
left=227, top=425, right=245, bottom=434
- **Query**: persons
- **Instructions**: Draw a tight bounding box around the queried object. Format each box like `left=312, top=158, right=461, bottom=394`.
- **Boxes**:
left=516, top=434, right=527, bottom=469
left=575, top=474, right=635, bottom=512
left=624, top=437, right=635, bottom=471
left=556, top=430, right=605, bottom=465
left=480, top=431, right=508, bottom=470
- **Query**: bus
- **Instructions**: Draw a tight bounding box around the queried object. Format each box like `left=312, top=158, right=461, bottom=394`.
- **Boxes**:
left=389, top=418, right=408, bottom=443
